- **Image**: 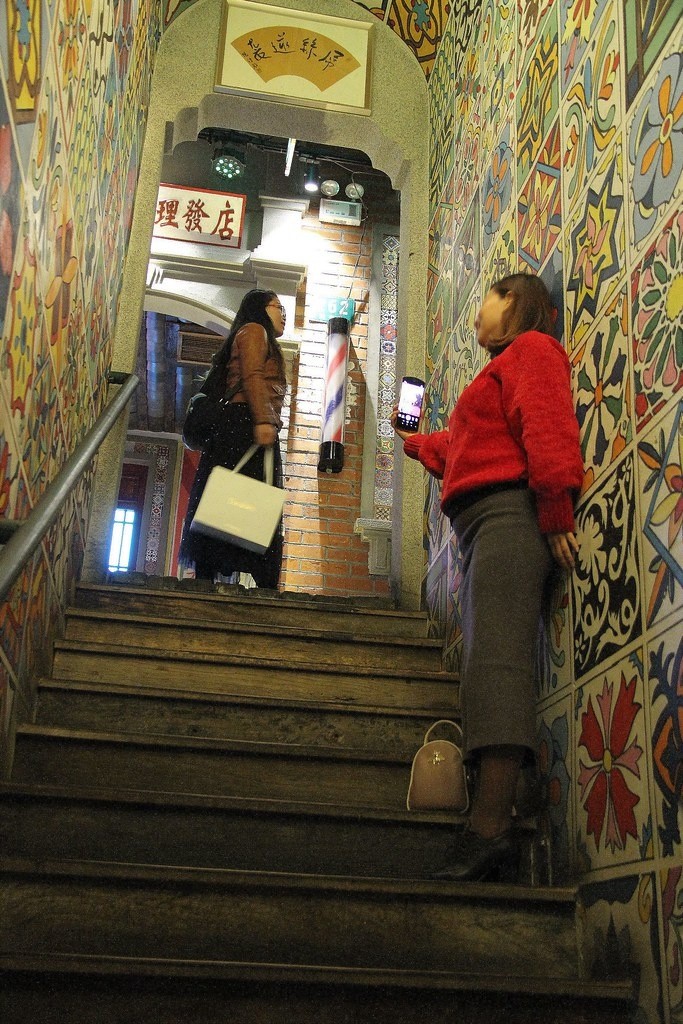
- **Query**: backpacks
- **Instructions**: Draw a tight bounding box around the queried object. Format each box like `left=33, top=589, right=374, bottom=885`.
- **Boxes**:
left=182, top=323, right=271, bottom=453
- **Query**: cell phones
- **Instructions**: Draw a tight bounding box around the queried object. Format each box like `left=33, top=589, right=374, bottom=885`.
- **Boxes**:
left=394, top=376, right=427, bottom=434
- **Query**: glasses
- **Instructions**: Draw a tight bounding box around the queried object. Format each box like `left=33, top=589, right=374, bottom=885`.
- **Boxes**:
left=265, top=304, right=287, bottom=319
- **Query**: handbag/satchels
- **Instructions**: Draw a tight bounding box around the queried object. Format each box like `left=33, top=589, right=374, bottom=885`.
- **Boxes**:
left=407, top=720, right=471, bottom=814
left=190, top=440, right=289, bottom=553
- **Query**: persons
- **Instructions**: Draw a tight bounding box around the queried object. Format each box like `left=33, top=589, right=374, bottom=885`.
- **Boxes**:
left=389, top=273, right=587, bottom=882
left=175, top=288, right=289, bottom=589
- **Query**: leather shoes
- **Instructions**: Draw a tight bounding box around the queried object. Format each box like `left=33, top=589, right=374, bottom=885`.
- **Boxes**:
left=432, top=828, right=527, bottom=885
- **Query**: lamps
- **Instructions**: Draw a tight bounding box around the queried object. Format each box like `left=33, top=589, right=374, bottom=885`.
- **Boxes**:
left=210, top=147, right=248, bottom=181
left=303, top=159, right=322, bottom=193
